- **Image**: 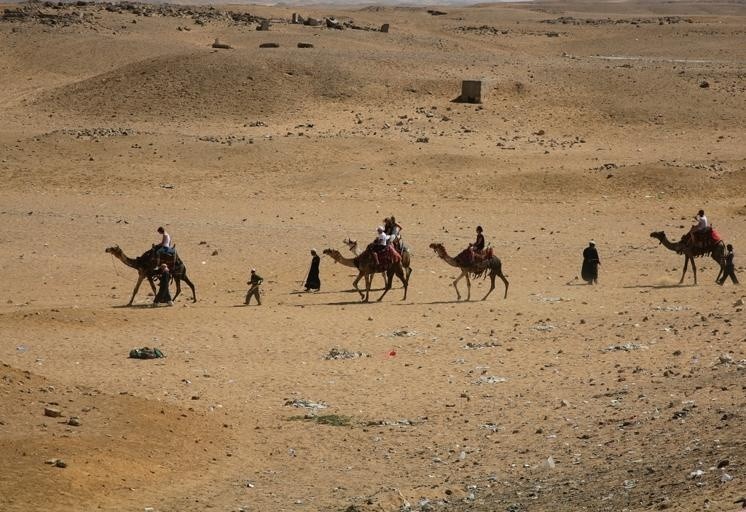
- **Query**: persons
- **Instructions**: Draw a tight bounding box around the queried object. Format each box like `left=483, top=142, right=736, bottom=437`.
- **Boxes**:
left=382, top=217, right=393, bottom=242
left=715, top=243, right=740, bottom=286
left=686, top=209, right=707, bottom=248
left=152, top=226, right=170, bottom=271
left=372, top=226, right=386, bottom=267
left=389, top=215, right=403, bottom=243
left=303, top=248, right=321, bottom=294
left=583, top=241, right=599, bottom=285
left=150, top=263, right=174, bottom=308
left=243, top=268, right=264, bottom=307
left=467, top=224, right=484, bottom=264
